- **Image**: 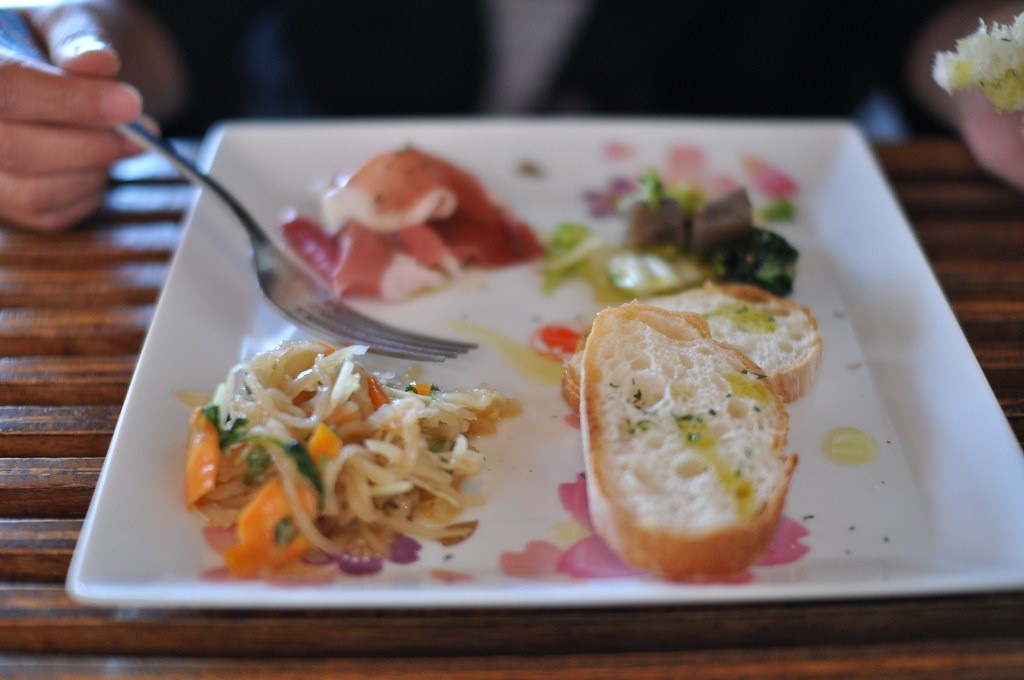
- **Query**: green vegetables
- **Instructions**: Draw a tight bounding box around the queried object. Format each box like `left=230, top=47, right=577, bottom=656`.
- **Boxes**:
left=543, top=175, right=797, bottom=296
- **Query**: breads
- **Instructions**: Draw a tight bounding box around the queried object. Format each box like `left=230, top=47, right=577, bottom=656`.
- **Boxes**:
left=932, top=10, right=1024, bottom=114
left=562, top=282, right=819, bottom=578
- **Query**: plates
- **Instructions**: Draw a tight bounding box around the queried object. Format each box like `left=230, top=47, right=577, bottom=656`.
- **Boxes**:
left=66, top=116, right=1024, bottom=609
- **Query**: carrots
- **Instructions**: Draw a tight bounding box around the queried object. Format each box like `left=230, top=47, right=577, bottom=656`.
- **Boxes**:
left=185, top=411, right=342, bottom=577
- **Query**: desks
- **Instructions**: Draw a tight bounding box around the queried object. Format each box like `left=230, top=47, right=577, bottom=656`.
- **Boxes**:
left=0, top=135, right=1024, bottom=679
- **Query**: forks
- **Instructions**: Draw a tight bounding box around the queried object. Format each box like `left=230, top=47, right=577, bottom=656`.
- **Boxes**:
left=0, top=6, right=481, bottom=363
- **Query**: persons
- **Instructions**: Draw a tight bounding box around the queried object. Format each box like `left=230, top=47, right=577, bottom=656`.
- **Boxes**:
left=0, top=0, right=1024, bottom=234
